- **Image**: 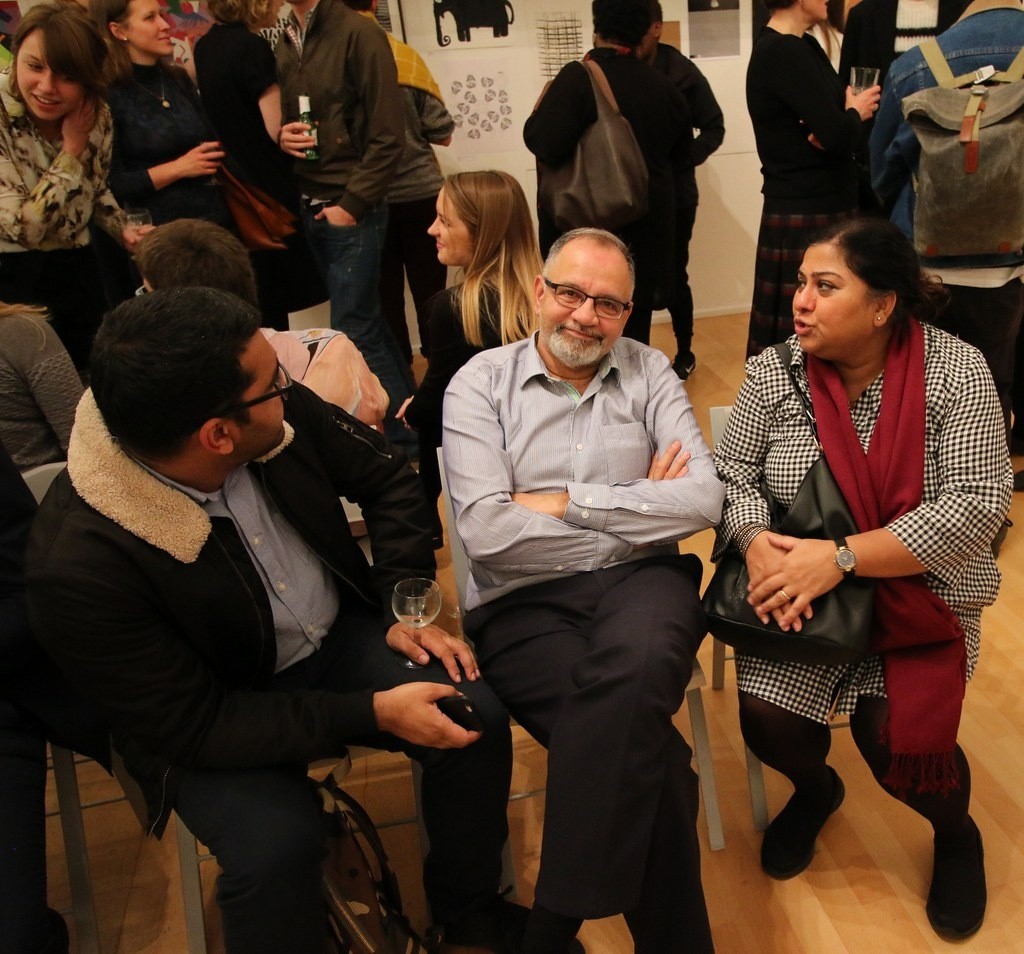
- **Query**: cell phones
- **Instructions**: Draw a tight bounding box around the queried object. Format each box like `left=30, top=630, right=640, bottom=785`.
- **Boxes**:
left=435, top=696, right=483, bottom=732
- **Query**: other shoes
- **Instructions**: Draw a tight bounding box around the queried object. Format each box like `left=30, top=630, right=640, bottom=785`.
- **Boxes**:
left=759, top=765, right=844, bottom=881
left=441, top=885, right=586, bottom=954
left=925, top=813, right=988, bottom=940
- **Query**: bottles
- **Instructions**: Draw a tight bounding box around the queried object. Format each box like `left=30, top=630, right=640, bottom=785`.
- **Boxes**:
left=298, top=90, right=321, bottom=162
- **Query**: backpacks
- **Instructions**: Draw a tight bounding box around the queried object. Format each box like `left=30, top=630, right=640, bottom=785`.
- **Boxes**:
left=899, top=35, right=1024, bottom=270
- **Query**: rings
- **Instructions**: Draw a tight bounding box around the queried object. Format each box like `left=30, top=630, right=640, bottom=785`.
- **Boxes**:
left=781, top=590, right=790, bottom=600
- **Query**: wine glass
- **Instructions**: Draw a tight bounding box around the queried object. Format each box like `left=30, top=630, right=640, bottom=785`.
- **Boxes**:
left=392, top=578, right=444, bottom=671
left=123, top=209, right=152, bottom=261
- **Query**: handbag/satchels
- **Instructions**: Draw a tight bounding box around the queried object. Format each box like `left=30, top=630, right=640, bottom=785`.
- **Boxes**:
left=703, top=462, right=879, bottom=663
left=217, top=163, right=301, bottom=254
left=534, top=57, right=650, bottom=239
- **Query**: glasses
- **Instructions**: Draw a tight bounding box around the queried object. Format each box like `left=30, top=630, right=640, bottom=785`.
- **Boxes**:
left=187, top=362, right=294, bottom=436
left=543, top=277, right=632, bottom=321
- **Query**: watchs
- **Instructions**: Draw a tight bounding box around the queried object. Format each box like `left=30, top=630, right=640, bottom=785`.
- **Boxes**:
left=833, top=537, right=856, bottom=578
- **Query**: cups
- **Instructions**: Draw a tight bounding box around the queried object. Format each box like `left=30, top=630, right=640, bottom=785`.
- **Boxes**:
left=850, top=67, right=881, bottom=94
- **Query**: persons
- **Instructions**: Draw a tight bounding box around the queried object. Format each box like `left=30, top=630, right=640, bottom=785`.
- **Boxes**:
left=0, top=286, right=583, bottom=954
left=523, top=0, right=725, bottom=380
left=713, top=215, right=1014, bottom=935
left=89, top=0, right=240, bottom=236
left=136, top=219, right=390, bottom=426
left=0, top=0, right=156, bottom=362
left=745, top=0, right=1024, bottom=495
left=0, top=301, right=85, bottom=470
left=397, top=171, right=545, bottom=549
left=190, top=0, right=282, bottom=193
left=442, top=228, right=726, bottom=954
left=275, top=0, right=419, bottom=461
left=343, top=0, right=455, bottom=395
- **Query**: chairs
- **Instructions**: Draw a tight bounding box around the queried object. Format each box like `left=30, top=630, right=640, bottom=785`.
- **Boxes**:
left=438, top=446, right=725, bottom=899
left=22, top=461, right=153, bottom=954
left=175, top=742, right=430, bottom=954
left=711, top=406, right=851, bottom=830
